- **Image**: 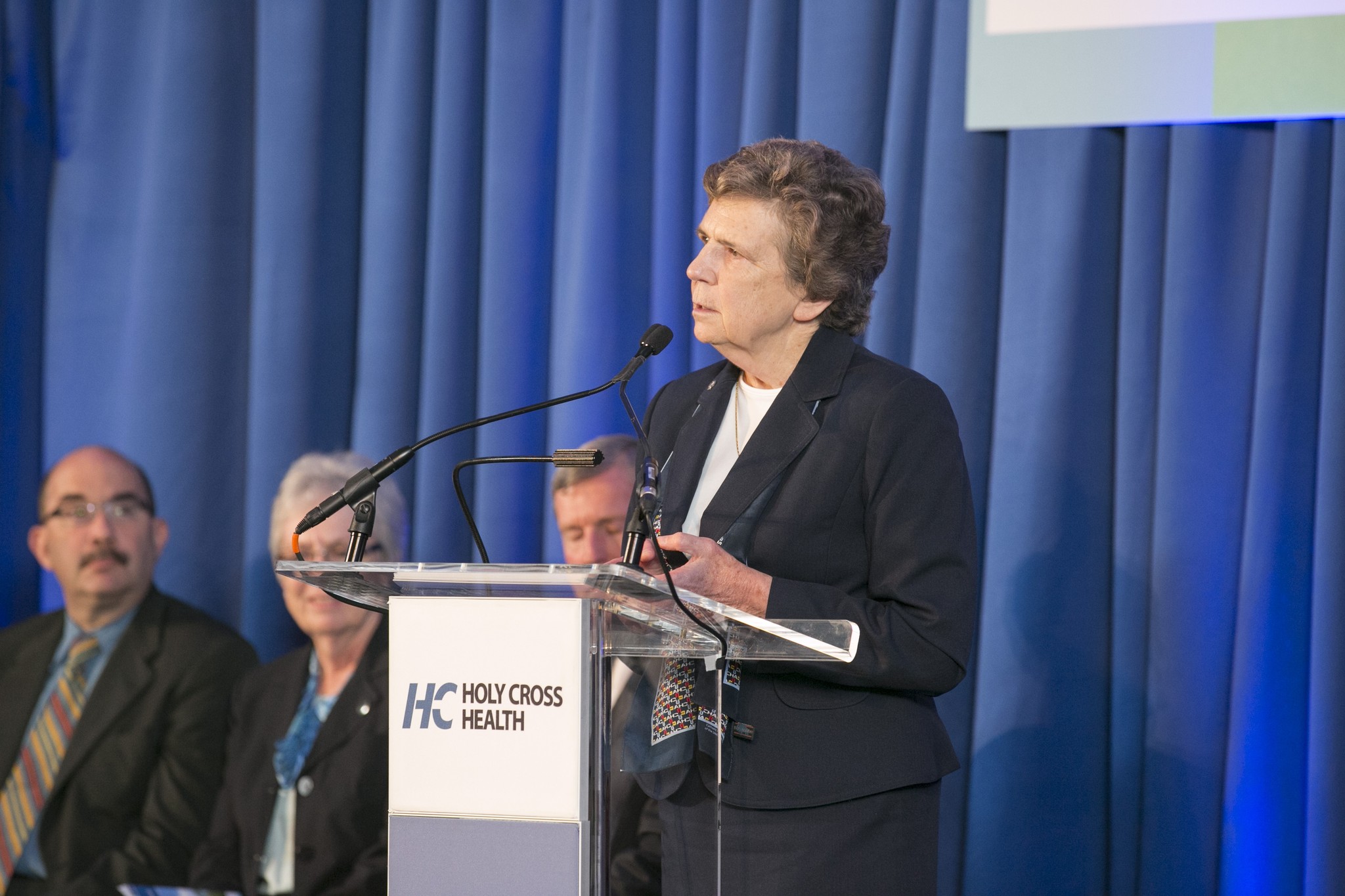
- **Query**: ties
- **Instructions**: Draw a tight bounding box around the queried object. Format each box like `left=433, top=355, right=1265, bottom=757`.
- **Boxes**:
left=2, top=630, right=103, bottom=896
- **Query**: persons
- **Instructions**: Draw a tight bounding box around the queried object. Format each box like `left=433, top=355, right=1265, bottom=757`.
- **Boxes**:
left=613, top=137, right=980, bottom=896
left=0, top=447, right=262, bottom=896
left=551, top=433, right=661, bottom=896
left=185, top=449, right=407, bottom=896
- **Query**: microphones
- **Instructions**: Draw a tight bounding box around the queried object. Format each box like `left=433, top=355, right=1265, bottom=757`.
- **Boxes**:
left=613, top=320, right=675, bottom=381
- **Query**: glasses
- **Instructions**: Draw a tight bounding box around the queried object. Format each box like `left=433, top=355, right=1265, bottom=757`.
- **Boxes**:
left=36, top=497, right=156, bottom=523
left=272, top=541, right=389, bottom=570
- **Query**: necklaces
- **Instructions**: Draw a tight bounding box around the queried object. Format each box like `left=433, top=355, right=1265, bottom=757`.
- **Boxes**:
left=735, top=373, right=739, bottom=458
